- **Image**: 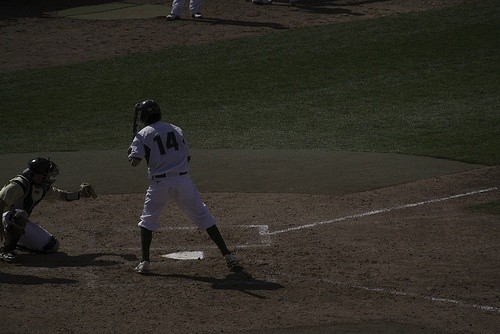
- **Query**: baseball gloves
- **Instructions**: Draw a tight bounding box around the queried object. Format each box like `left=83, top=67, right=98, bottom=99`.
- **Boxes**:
left=78, top=181, right=97, bottom=199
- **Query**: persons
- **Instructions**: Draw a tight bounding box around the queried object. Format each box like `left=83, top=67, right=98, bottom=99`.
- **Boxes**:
left=0, top=157, right=97, bottom=262
left=126, top=100, right=244, bottom=274
left=167, top=0, right=203, bottom=21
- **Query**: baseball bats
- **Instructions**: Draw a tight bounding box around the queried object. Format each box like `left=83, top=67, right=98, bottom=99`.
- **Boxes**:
left=132, top=102, right=142, bottom=169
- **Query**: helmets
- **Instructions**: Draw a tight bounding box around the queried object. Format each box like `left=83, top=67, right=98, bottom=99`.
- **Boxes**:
left=28, top=157, right=59, bottom=177
left=140, top=100, right=159, bottom=112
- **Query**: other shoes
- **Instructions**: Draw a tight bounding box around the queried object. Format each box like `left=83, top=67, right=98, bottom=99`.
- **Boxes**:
left=226, top=256, right=244, bottom=271
left=166, top=13, right=180, bottom=20
left=193, top=13, right=202, bottom=18
left=0, top=247, right=18, bottom=262
left=133, top=261, right=150, bottom=274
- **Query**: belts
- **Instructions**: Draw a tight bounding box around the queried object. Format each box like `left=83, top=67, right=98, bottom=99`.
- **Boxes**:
left=152, top=172, right=187, bottom=180
